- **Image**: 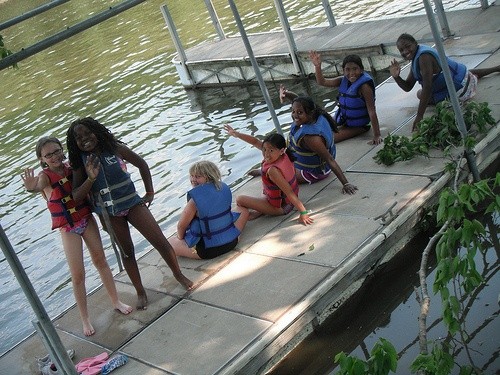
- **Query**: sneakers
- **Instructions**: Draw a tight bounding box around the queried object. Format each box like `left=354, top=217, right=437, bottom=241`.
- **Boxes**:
left=35, top=349, right=75, bottom=375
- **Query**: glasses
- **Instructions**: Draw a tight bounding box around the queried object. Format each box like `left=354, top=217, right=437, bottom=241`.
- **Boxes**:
left=41, top=149, right=62, bottom=159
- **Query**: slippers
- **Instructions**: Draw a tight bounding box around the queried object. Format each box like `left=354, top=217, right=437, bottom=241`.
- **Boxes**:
left=74, top=352, right=127, bottom=375
